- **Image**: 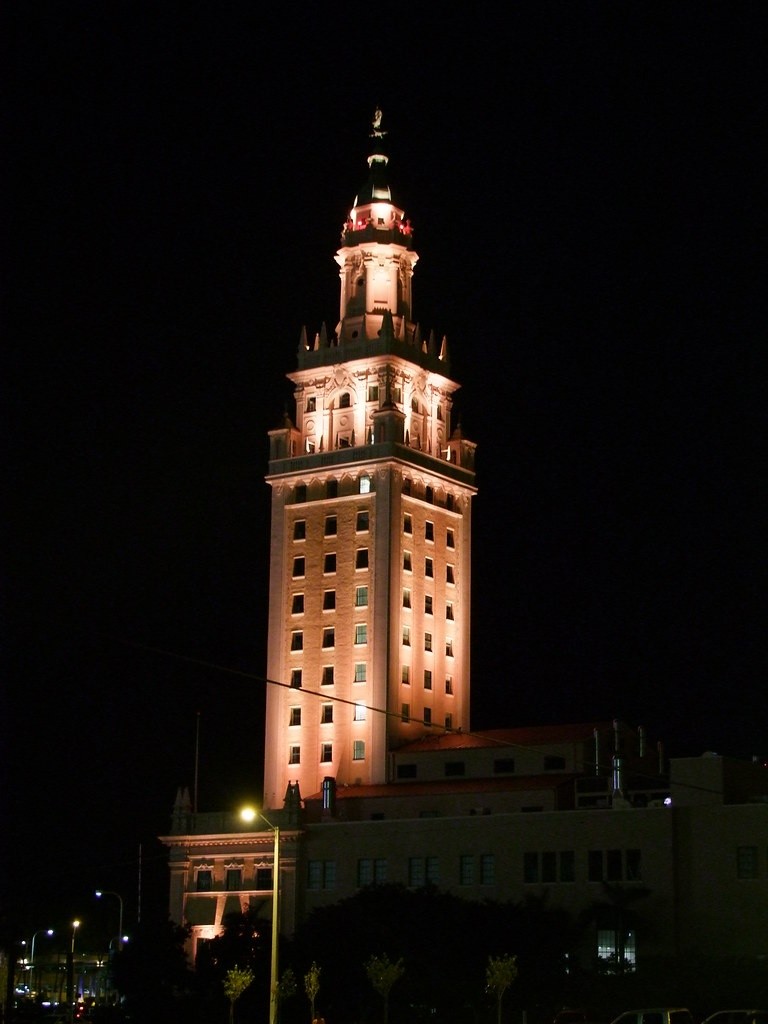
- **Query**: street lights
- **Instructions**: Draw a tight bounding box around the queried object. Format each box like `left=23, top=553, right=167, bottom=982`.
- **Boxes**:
left=237, top=805, right=281, bottom=1023
left=29, top=929, right=54, bottom=1000
left=71, top=920, right=80, bottom=953
left=95, top=890, right=124, bottom=952
left=104, top=935, right=129, bottom=1004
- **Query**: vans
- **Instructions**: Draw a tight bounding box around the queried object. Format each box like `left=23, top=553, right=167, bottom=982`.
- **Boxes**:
left=612, top=1007, right=695, bottom=1024
left=700, top=1008, right=765, bottom=1024
